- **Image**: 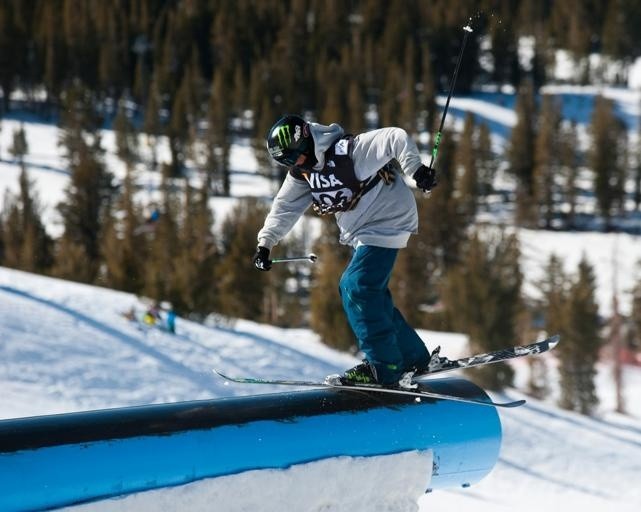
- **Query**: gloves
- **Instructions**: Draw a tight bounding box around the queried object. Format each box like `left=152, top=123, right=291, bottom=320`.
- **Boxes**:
left=412, top=165, right=437, bottom=190
left=253, top=247, right=272, bottom=271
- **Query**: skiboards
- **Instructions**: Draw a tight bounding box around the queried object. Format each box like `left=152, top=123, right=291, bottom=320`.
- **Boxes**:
left=215, top=335, right=559, bottom=408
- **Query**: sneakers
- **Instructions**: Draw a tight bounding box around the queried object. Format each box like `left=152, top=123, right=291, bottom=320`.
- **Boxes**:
left=325, top=363, right=414, bottom=386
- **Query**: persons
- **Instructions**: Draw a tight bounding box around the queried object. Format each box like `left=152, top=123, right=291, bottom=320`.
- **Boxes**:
left=254, top=113, right=438, bottom=390
left=121, top=300, right=178, bottom=335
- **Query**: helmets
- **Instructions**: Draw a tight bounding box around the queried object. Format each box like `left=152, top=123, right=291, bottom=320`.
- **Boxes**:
left=267, top=116, right=316, bottom=170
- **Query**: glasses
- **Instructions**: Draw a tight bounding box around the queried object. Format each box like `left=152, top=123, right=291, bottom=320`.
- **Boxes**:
left=274, top=151, right=300, bottom=167
